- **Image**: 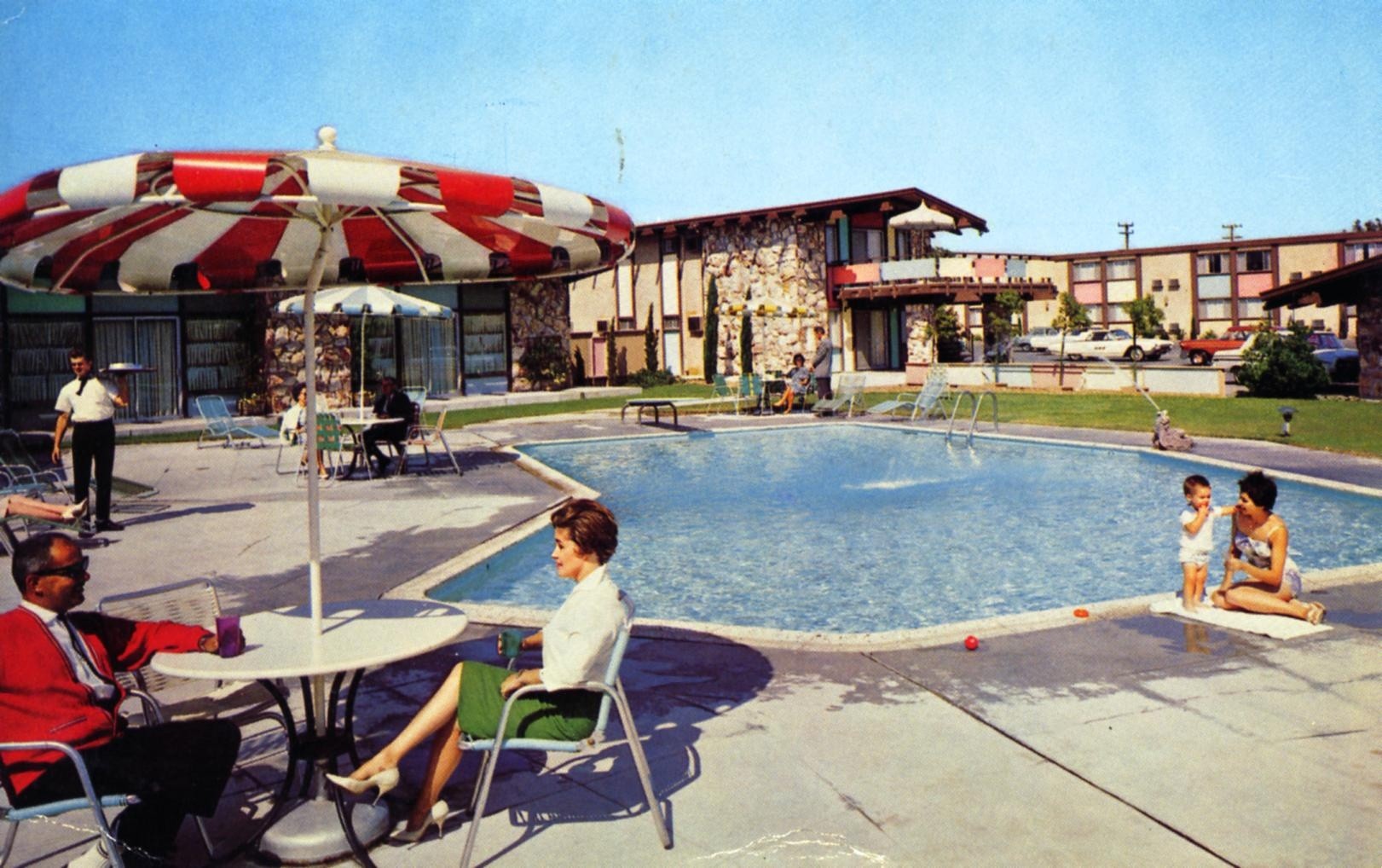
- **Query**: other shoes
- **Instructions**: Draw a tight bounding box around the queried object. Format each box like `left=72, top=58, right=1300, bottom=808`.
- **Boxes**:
left=300, top=460, right=309, bottom=470
left=79, top=524, right=91, bottom=537
left=377, top=457, right=391, bottom=474
left=96, top=519, right=123, bottom=531
left=98, top=815, right=176, bottom=868
left=365, top=461, right=373, bottom=471
left=784, top=408, right=791, bottom=413
left=773, top=398, right=786, bottom=405
left=1300, top=601, right=1325, bottom=623
left=318, top=468, right=328, bottom=479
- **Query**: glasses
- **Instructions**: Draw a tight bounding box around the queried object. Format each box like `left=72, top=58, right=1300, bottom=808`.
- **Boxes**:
left=33, top=556, right=88, bottom=578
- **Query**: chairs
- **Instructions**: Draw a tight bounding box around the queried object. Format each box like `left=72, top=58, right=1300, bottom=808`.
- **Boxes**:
left=457, top=590, right=672, bottom=867
left=867, top=371, right=947, bottom=425
left=96, top=578, right=293, bottom=867
left=705, top=371, right=815, bottom=417
left=0, top=689, right=219, bottom=868
left=1, top=464, right=97, bottom=559
left=275, top=385, right=462, bottom=488
left=812, top=373, right=867, bottom=421
left=195, top=395, right=280, bottom=449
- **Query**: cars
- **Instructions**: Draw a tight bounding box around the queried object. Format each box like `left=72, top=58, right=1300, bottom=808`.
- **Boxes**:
left=1178, top=327, right=1360, bottom=385
left=1014, top=324, right=1174, bottom=362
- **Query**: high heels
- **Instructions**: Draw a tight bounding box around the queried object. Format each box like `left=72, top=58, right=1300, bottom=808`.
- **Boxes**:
left=390, top=799, right=449, bottom=842
left=326, top=768, right=400, bottom=808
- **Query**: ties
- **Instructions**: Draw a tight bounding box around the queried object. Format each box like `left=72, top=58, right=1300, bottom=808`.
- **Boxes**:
left=57, top=613, right=117, bottom=687
left=387, top=396, right=391, bottom=410
left=77, top=377, right=90, bottom=395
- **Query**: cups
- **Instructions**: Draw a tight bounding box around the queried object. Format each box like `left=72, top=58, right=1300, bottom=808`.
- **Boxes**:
left=215, top=616, right=239, bottom=659
left=502, top=630, right=520, bottom=658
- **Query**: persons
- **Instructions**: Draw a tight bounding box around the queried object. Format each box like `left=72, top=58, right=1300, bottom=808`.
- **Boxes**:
left=0, top=531, right=242, bottom=868
left=281, top=384, right=329, bottom=480
left=773, top=353, right=811, bottom=414
left=360, top=376, right=416, bottom=477
left=1208, top=470, right=1327, bottom=625
left=52, top=347, right=130, bottom=537
left=810, top=326, right=834, bottom=417
left=325, top=496, right=626, bottom=843
left=1179, top=475, right=1240, bottom=613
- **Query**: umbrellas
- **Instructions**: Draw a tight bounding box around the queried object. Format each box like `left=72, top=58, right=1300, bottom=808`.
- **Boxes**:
left=271, top=284, right=456, bottom=420
left=0, top=126, right=635, bottom=801
left=889, top=197, right=955, bottom=258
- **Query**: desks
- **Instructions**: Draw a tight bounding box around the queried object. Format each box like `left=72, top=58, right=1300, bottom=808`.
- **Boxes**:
left=330, top=416, right=404, bottom=476
left=151, top=600, right=468, bottom=868
left=623, top=400, right=677, bottom=430
